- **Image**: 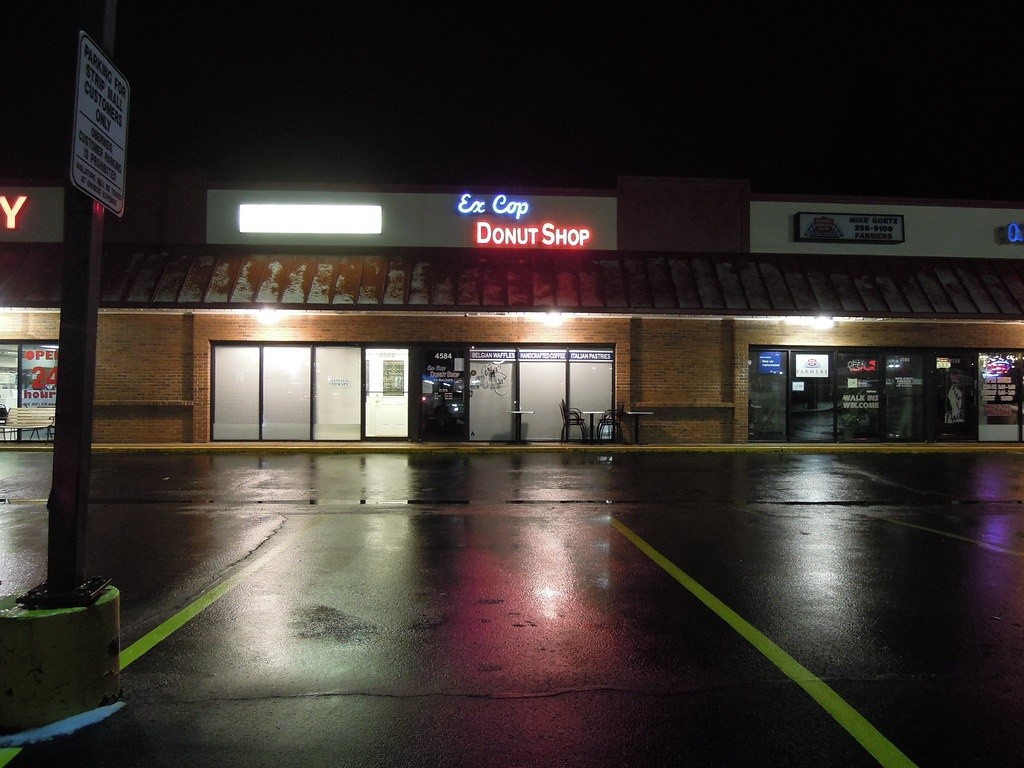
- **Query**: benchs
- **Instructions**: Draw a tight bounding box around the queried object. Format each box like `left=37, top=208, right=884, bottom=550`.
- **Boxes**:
left=1, top=408, right=57, bottom=445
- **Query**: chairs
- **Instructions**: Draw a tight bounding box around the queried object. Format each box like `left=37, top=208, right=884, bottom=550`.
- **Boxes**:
left=559, top=404, right=585, bottom=445
left=0, top=404, right=7, bottom=440
left=561, top=399, right=584, bottom=442
left=599, top=404, right=625, bottom=442
left=597, top=404, right=622, bottom=441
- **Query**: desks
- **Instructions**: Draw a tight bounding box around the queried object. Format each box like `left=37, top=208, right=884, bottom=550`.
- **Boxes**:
left=507, top=411, right=534, bottom=444
left=626, top=411, right=654, bottom=445
left=582, top=411, right=602, bottom=444
